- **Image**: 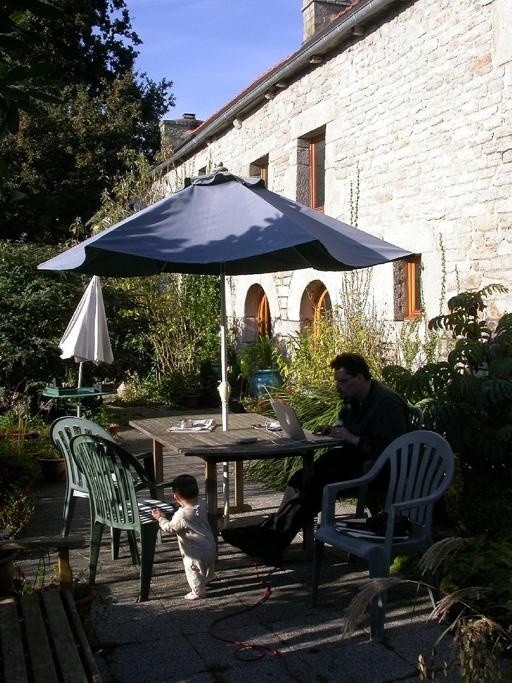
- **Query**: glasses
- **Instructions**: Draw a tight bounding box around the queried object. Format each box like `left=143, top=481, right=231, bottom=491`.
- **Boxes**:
left=335, top=378, right=353, bottom=384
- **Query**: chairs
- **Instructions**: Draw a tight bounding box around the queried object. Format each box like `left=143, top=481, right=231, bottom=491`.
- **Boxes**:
left=311, top=429, right=456, bottom=646
left=48, top=415, right=173, bottom=566
left=69, top=433, right=180, bottom=603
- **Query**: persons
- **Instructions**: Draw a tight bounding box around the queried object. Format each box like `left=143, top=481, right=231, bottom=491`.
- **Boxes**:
left=151, top=474, right=216, bottom=599
left=223, top=352, right=412, bottom=566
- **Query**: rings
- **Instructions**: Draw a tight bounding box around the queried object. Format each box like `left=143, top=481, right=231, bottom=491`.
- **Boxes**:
left=336, top=430, right=340, bottom=433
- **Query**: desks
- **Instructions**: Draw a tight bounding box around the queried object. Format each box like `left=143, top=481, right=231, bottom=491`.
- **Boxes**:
left=129, top=412, right=345, bottom=561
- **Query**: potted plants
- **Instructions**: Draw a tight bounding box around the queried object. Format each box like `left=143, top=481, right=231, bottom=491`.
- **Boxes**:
left=0, top=404, right=121, bottom=487
left=195, top=326, right=244, bottom=404
left=250, top=332, right=284, bottom=401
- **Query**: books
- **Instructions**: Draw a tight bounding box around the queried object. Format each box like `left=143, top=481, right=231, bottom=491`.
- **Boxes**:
left=166, top=418, right=217, bottom=433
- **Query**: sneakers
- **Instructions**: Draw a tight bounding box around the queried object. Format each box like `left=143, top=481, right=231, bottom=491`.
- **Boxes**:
left=221, top=525, right=288, bottom=562
left=184, top=591, right=208, bottom=601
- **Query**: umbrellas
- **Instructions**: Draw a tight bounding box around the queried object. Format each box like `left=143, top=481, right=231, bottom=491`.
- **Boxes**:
left=57, top=274, right=116, bottom=416
left=37, top=161, right=422, bottom=428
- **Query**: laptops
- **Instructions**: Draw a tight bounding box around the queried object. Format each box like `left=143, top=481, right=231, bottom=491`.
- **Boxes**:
left=270, top=401, right=338, bottom=443
